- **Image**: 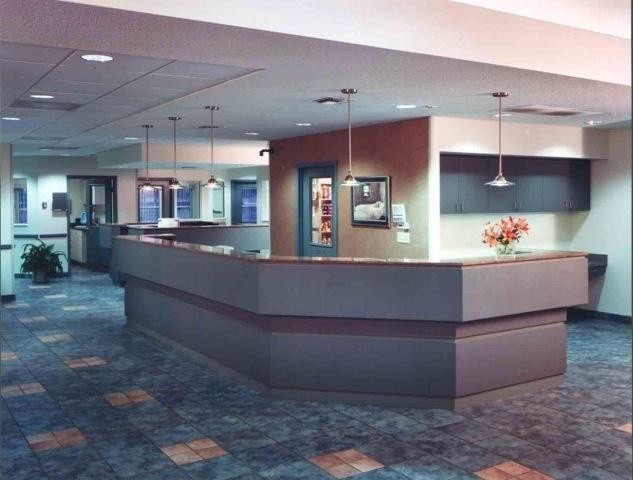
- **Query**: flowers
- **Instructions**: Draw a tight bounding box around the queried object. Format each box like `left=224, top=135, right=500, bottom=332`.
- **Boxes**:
left=482, top=215, right=530, bottom=247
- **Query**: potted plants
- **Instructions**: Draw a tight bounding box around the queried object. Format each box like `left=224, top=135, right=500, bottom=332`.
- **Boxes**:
left=20, top=238, right=68, bottom=285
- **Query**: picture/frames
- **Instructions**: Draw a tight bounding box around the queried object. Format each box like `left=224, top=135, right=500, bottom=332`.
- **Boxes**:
left=351, top=176, right=391, bottom=228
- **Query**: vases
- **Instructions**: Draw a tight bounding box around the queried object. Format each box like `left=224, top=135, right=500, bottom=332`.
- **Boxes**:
left=494, top=242, right=516, bottom=261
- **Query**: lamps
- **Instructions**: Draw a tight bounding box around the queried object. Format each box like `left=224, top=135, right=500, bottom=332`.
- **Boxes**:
left=340, top=89, right=360, bottom=186
left=162, top=117, right=187, bottom=190
left=136, top=125, right=158, bottom=190
left=483, top=92, right=517, bottom=187
left=199, top=106, right=227, bottom=188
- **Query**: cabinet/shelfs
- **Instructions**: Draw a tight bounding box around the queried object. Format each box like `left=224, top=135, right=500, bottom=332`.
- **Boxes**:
left=138, top=185, right=162, bottom=223
left=318, top=183, right=332, bottom=244
left=240, top=187, right=257, bottom=223
left=440, top=155, right=590, bottom=214
left=174, top=181, right=200, bottom=219
